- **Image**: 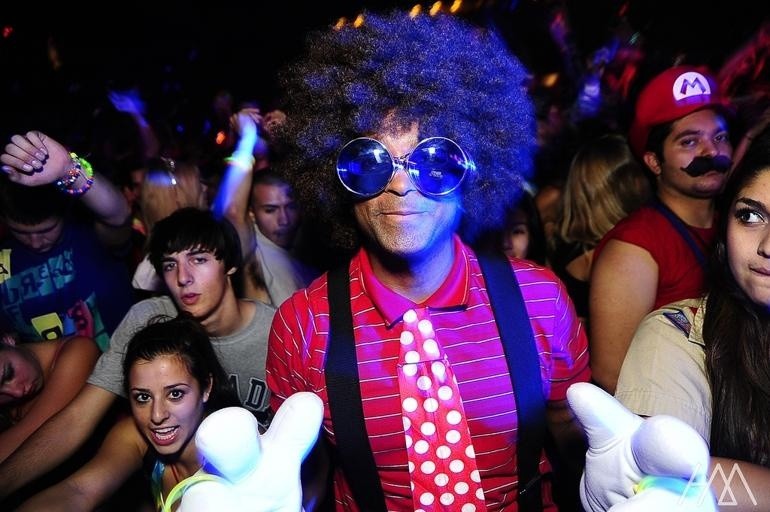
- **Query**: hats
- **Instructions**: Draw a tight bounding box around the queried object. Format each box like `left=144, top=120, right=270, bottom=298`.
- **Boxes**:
left=636, top=66, right=734, bottom=127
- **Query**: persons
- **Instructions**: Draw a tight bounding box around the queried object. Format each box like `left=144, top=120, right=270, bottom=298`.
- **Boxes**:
left=1, top=7, right=768, bottom=511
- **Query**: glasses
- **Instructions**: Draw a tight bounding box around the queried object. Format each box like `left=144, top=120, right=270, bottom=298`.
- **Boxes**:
left=335, top=135, right=476, bottom=198
